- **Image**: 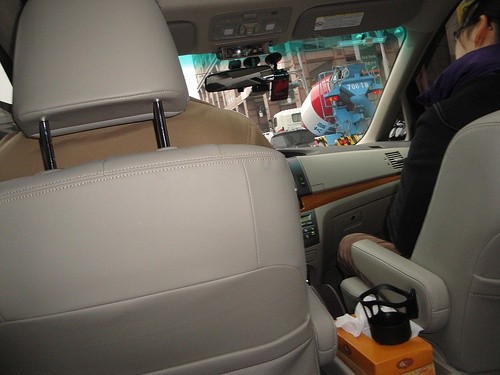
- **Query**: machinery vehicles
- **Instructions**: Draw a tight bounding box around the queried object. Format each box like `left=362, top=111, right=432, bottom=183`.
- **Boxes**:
left=269, top=60, right=384, bottom=146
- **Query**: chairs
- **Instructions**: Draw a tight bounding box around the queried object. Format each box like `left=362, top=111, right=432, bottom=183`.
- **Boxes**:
left=0, top=0, right=336, bottom=375
left=339, top=111, right=499, bottom=373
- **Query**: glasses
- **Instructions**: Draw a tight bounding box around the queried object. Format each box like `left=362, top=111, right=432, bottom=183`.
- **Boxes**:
left=453, top=19, right=494, bottom=39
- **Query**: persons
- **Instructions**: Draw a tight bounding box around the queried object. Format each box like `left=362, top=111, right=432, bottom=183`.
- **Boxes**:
left=336, top=0, right=500, bottom=280
